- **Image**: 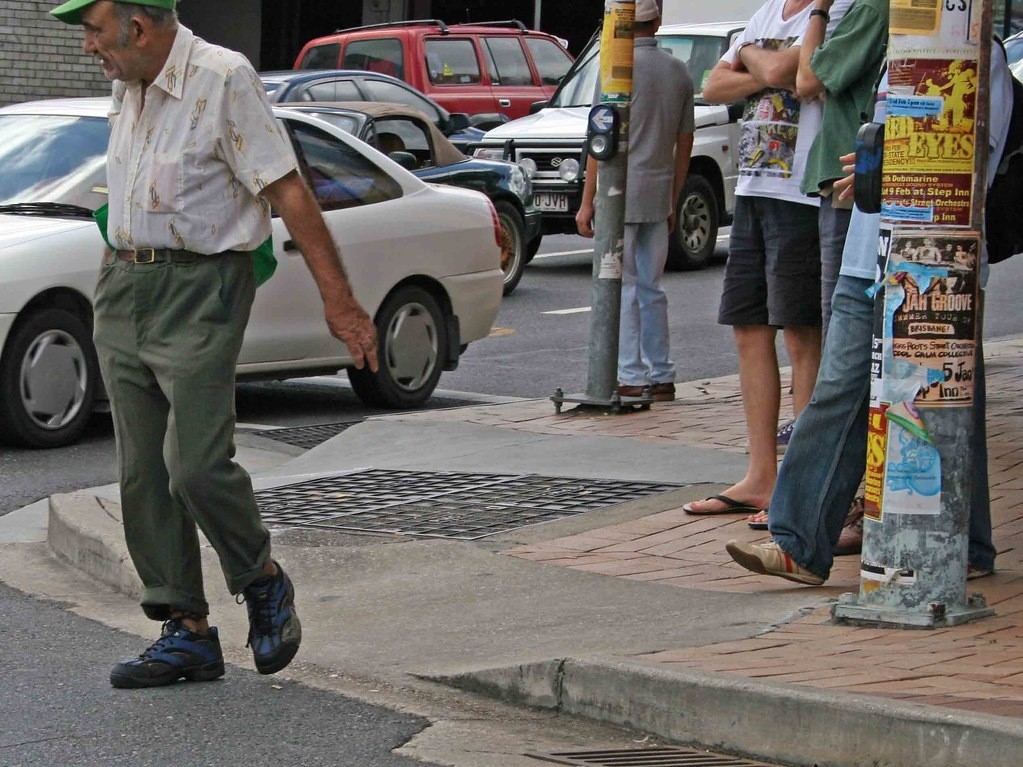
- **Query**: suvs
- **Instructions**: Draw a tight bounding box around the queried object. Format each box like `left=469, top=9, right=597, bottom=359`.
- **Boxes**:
left=471, top=18, right=749, bottom=274
left=292, top=19, right=578, bottom=131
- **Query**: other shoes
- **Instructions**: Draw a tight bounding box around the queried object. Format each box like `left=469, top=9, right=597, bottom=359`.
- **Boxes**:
left=831, top=498, right=866, bottom=554
left=615, top=381, right=677, bottom=403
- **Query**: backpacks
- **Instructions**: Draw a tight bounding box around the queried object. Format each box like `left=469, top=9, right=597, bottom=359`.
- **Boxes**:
left=984, top=33, right=1023, bottom=264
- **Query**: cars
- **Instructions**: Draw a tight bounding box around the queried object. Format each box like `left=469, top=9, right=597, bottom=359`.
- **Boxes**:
left=254, top=68, right=544, bottom=297
left=1, top=96, right=503, bottom=451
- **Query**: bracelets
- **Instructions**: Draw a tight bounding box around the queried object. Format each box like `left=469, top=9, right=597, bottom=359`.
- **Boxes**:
left=809, top=9, right=830, bottom=22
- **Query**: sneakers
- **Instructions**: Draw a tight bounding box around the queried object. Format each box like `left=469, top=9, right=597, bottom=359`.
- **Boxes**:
left=773, top=419, right=802, bottom=454
left=726, top=540, right=826, bottom=585
left=237, top=562, right=301, bottom=675
left=109, top=615, right=226, bottom=688
left=967, top=566, right=994, bottom=579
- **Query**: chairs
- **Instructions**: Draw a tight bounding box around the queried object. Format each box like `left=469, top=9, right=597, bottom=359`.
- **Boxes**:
left=685, top=56, right=713, bottom=94
left=367, top=132, right=405, bottom=156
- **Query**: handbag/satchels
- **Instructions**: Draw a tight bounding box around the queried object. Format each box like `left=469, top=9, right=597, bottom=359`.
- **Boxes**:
left=93, top=198, right=278, bottom=288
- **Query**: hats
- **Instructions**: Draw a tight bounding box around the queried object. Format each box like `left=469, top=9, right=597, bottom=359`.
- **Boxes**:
left=632, top=0, right=658, bottom=22
left=49, top=0, right=176, bottom=27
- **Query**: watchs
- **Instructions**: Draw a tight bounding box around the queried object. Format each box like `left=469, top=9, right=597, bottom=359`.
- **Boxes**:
left=738, top=41, right=756, bottom=54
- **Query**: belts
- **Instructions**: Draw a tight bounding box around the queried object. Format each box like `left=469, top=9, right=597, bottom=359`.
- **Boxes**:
left=114, top=247, right=201, bottom=265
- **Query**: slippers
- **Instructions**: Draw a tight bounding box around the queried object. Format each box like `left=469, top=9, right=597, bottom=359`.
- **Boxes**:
left=748, top=505, right=770, bottom=528
left=681, top=495, right=766, bottom=516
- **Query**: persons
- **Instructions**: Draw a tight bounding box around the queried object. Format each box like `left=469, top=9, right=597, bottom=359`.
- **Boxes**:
left=575, top=0, right=695, bottom=402
left=50, top=0, right=378, bottom=689
left=683, top=0, right=1023, bottom=585
